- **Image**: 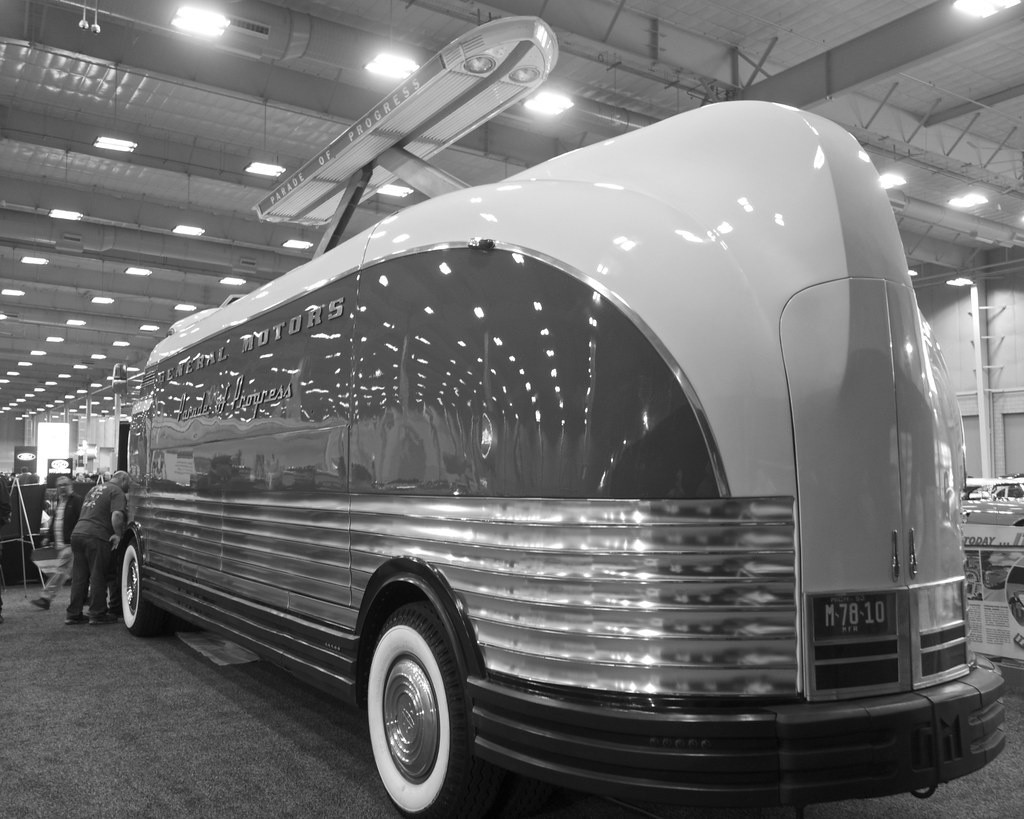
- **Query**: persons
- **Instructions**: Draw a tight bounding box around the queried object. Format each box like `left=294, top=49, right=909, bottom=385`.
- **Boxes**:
left=18, top=467, right=40, bottom=484
left=197, top=456, right=256, bottom=567
left=64, top=470, right=130, bottom=625
left=31, top=477, right=83, bottom=610
left=0, top=471, right=16, bottom=486
left=76, top=473, right=84, bottom=482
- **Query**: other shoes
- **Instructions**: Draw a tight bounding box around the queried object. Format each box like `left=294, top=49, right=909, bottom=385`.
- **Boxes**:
left=84, top=596, right=91, bottom=605
left=31, top=597, right=50, bottom=610
left=89, top=613, right=118, bottom=624
left=65, top=612, right=89, bottom=625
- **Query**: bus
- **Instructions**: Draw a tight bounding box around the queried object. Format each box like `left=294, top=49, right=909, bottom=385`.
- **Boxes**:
left=109, top=15, right=1012, bottom=819
